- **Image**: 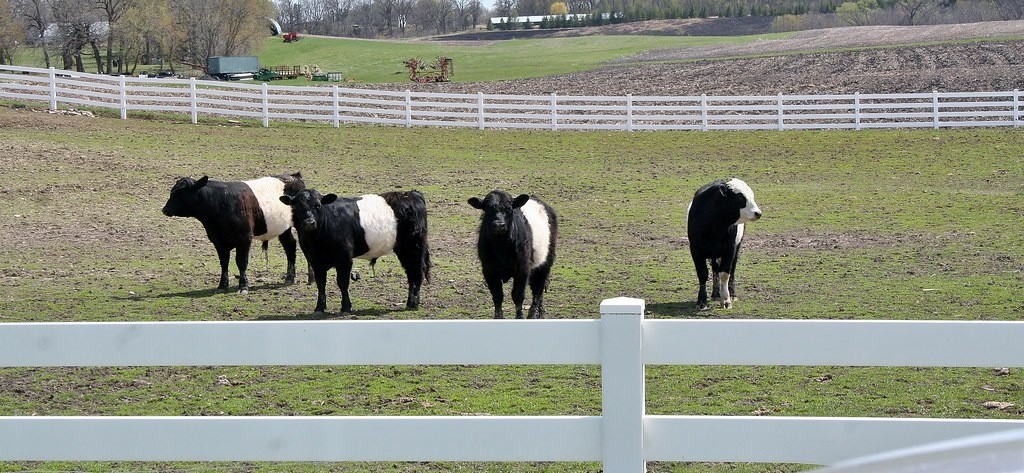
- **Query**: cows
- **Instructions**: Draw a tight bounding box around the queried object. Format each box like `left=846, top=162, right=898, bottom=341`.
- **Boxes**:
left=161, top=171, right=431, bottom=316
left=468, top=191, right=557, bottom=321
left=686, top=177, right=762, bottom=311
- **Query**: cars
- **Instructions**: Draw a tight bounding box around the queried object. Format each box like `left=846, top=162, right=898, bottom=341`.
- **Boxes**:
left=140, top=70, right=155, bottom=78
